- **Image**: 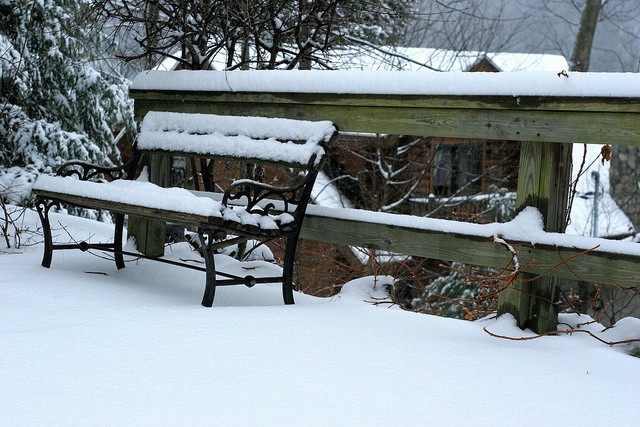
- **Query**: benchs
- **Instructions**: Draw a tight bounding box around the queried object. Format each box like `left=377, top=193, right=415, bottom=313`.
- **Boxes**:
left=29, top=108, right=339, bottom=308
